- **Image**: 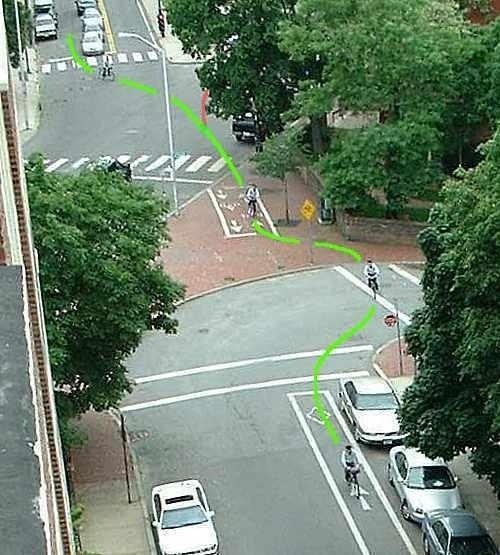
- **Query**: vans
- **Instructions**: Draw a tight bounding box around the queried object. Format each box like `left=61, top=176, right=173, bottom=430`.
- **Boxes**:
left=232, top=105, right=267, bottom=143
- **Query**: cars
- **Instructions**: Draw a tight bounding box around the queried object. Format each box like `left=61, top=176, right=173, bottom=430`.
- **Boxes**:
left=337, top=376, right=409, bottom=447
left=151, top=479, right=219, bottom=555
left=387, top=445, right=463, bottom=523
left=422, top=509, right=499, bottom=555
left=91, top=158, right=132, bottom=183
left=32, top=0, right=104, bottom=55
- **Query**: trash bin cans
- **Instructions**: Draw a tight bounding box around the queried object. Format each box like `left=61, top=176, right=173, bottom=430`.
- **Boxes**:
left=318, top=197, right=334, bottom=224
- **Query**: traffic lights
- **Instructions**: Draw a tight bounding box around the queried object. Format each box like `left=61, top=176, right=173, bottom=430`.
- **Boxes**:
left=157, top=12, right=165, bottom=34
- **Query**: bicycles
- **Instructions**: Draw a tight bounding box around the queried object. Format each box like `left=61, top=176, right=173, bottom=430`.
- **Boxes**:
left=344, top=465, right=360, bottom=499
left=247, top=196, right=259, bottom=216
left=367, top=273, right=379, bottom=299
left=96, top=63, right=115, bottom=81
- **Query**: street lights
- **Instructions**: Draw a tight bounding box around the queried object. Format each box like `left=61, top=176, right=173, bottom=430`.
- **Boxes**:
left=118, top=32, right=180, bottom=218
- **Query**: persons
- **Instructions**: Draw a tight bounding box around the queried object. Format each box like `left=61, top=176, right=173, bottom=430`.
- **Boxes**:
left=341, top=445, right=358, bottom=485
left=103, top=54, right=113, bottom=74
left=246, top=182, right=257, bottom=211
left=365, top=259, right=379, bottom=290
